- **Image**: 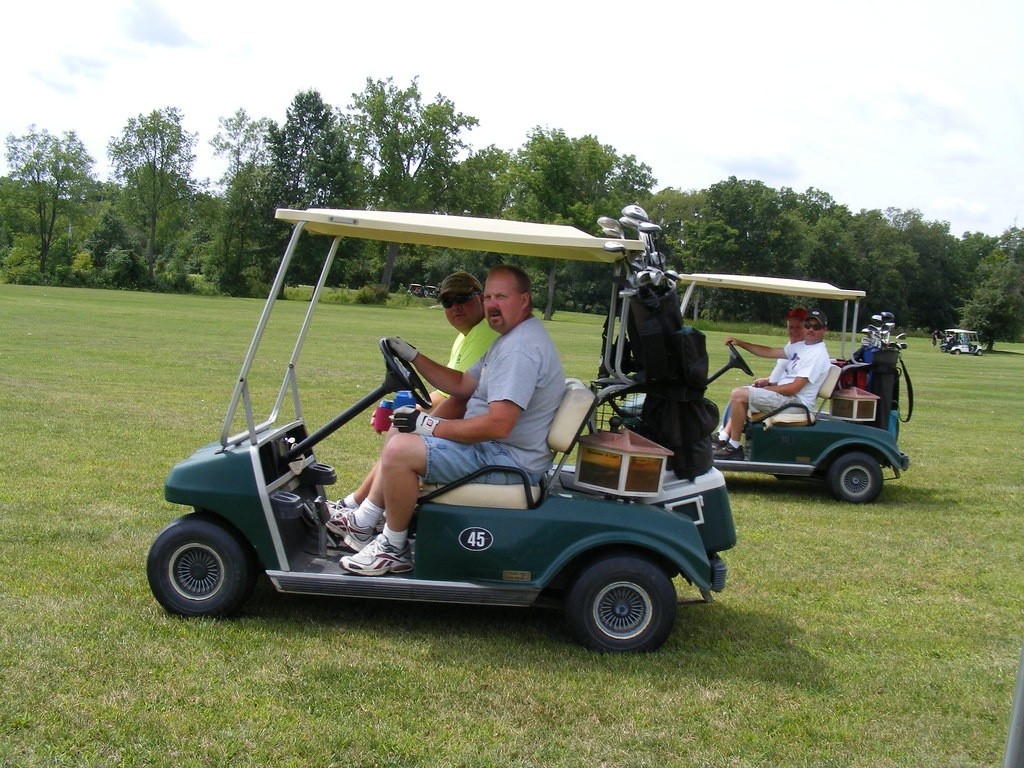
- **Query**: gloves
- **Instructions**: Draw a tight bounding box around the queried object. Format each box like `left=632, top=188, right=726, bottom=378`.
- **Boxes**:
left=387, top=336, right=419, bottom=362
left=391, top=407, right=440, bottom=437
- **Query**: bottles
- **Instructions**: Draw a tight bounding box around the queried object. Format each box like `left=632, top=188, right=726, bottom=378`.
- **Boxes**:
left=373, top=399, right=393, bottom=431
left=393, top=390, right=416, bottom=409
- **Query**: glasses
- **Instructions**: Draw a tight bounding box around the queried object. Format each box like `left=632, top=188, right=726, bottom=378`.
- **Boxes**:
left=440, top=291, right=478, bottom=308
left=804, top=324, right=824, bottom=331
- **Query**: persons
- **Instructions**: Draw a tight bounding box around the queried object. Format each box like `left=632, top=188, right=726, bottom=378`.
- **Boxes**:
left=710, top=307, right=831, bottom=460
left=321, top=264, right=567, bottom=577
left=324, top=270, right=502, bottom=577
left=932, top=329, right=938, bottom=348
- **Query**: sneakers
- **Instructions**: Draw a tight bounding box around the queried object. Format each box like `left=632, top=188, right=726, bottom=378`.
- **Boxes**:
left=714, top=442, right=745, bottom=460
left=344, top=533, right=379, bottom=553
left=324, top=500, right=356, bottom=517
left=711, top=432, right=729, bottom=448
left=338, top=534, right=414, bottom=576
left=325, top=515, right=373, bottom=540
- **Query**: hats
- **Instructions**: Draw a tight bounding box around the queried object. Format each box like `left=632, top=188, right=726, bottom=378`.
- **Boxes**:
left=785, top=308, right=807, bottom=321
left=805, top=308, right=827, bottom=325
left=437, top=271, right=482, bottom=304
